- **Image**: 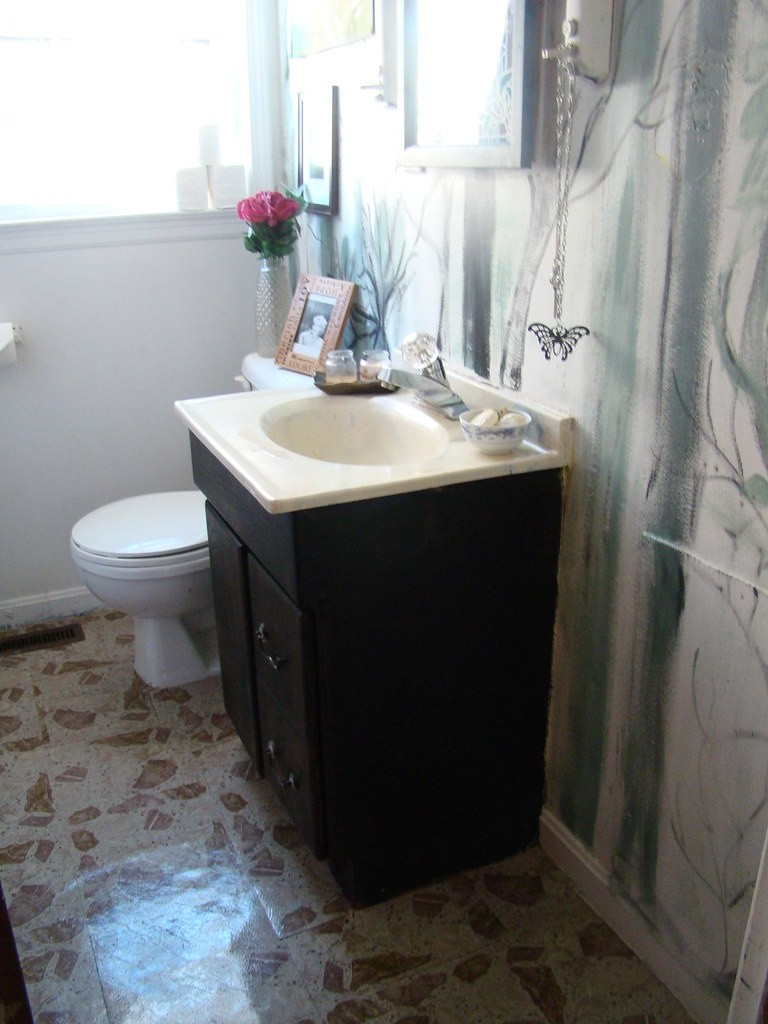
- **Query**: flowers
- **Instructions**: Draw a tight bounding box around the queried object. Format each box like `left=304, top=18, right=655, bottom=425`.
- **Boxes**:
left=236, top=184, right=307, bottom=260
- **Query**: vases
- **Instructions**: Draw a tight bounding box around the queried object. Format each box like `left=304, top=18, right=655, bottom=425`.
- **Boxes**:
left=255, top=259, right=293, bottom=359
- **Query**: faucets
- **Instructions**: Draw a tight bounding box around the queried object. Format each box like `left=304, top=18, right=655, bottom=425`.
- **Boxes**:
left=377, top=366, right=470, bottom=421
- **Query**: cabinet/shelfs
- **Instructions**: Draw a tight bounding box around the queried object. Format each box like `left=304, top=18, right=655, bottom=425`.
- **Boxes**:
left=189, top=430, right=563, bottom=911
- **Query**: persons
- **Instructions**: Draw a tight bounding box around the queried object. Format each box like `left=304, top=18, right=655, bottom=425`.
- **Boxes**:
left=301, top=315, right=328, bottom=349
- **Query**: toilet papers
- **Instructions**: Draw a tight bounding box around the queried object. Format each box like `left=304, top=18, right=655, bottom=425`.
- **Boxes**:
left=0, top=322, right=17, bottom=367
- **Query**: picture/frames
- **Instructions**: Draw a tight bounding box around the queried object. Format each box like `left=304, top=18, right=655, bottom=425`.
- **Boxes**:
left=298, top=84, right=341, bottom=217
left=273, top=272, right=359, bottom=376
left=289, top=0, right=377, bottom=59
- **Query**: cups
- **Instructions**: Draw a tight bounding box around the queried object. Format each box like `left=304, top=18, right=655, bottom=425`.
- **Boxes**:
left=359, top=349, right=391, bottom=384
left=325, top=349, right=357, bottom=385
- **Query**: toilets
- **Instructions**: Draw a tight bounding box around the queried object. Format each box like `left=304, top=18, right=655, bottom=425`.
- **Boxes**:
left=70, top=353, right=314, bottom=689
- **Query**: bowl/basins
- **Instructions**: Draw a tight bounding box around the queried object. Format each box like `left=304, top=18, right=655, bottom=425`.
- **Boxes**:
left=459, top=408, right=531, bottom=455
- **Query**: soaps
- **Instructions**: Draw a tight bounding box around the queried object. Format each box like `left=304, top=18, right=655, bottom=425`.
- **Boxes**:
left=468, top=409, right=525, bottom=427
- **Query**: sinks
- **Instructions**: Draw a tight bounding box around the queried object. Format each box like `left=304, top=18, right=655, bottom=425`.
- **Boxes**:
left=259, top=393, right=447, bottom=467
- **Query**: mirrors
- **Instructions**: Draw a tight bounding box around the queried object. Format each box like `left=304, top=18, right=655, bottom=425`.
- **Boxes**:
left=396, top=0, right=537, bottom=169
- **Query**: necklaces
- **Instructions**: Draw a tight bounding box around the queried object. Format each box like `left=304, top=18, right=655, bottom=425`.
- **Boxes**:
left=528, top=45, right=589, bottom=362
